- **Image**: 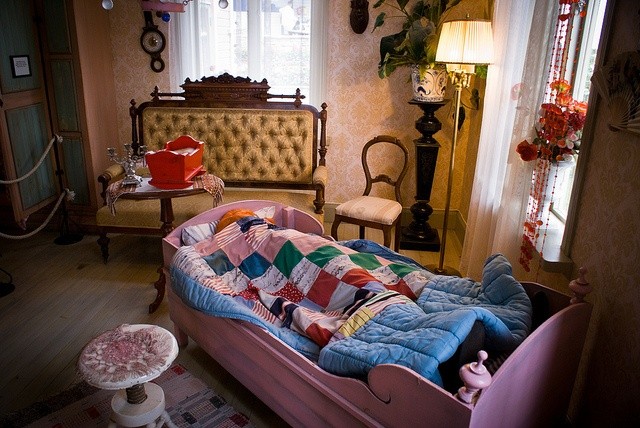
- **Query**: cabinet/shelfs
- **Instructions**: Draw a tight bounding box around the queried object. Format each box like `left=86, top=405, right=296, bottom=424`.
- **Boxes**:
left=0, top=1, right=60, bottom=227
left=33, top=0, right=121, bottom=228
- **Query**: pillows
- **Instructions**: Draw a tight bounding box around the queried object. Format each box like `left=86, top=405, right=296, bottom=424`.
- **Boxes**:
left=181, top=206, right=276, bottom=246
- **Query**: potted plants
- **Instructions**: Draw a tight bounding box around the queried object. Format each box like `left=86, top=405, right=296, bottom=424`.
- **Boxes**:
left=371, top=0, right=490, bottom=132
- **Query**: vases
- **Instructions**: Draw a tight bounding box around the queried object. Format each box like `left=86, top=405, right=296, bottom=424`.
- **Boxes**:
left=534, top=155, right=575, bottom=199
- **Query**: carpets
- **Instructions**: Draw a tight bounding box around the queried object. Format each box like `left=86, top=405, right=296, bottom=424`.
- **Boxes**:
left=0, top=361, right=260, bottom=427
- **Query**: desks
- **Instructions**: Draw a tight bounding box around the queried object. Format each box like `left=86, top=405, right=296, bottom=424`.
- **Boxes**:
left=107, top=178, right=228, bottom=313
left=76, top=324, right=179, bottom=428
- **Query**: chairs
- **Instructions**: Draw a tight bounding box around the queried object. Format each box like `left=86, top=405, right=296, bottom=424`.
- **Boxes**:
left=332, top=136, right=410, bottom=252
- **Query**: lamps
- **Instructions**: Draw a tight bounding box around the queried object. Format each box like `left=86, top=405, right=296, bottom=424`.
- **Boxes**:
left=423, top=15, right=494, bottom=277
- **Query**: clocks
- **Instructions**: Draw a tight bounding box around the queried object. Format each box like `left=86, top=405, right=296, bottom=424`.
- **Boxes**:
left=141, top=12, right=167, bottom=73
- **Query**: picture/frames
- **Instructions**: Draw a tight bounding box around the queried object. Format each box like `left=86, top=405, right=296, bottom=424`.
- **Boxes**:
left=9, top=54, right=33, bottom=77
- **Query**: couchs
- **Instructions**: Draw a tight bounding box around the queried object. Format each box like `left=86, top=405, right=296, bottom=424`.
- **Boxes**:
left=88, top=74, right=328, bottom=263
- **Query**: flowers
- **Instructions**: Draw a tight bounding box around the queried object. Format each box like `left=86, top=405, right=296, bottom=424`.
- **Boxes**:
left=515, top=81, right=590, bottom=165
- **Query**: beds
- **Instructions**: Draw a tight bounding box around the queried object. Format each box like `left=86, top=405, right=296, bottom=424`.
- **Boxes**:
left=160, top=199, right=594, bottom=428
